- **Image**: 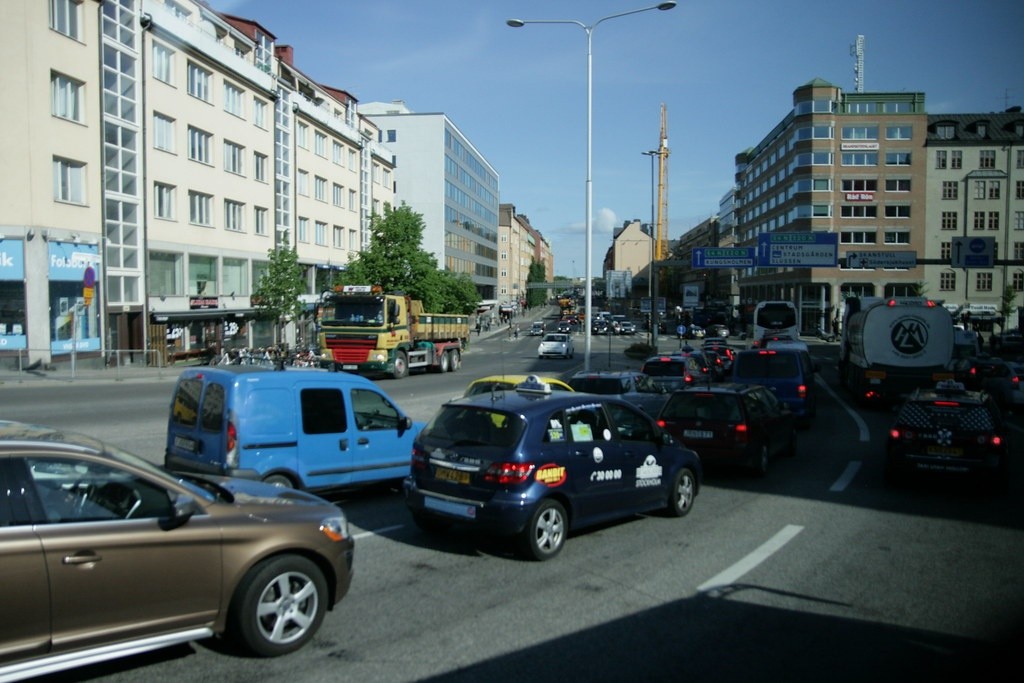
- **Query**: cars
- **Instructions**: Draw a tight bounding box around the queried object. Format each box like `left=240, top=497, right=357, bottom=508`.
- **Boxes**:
left=639, top=334, right=821, bottom=428
left=410, top=387, right=701, bottom=554
left=883, top=378, right=1012, bottom=492
left=657, top=383, right=796, bottom=477
left=563, top=370, right=671, bottom=420
left=1, top=418, right=354, bottom=674
left=161, top=367, right=426, bottom=496
left=527, top=310, right=729, bottom=358
left=931, top=326, right=1024, bottom=404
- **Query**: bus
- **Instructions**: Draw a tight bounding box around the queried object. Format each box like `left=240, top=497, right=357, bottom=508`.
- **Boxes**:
left=751, top=299, right=803, bottom=352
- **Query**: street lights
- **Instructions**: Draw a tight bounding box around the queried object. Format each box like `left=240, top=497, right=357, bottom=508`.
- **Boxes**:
left=506, top=0, right=683, bottom=374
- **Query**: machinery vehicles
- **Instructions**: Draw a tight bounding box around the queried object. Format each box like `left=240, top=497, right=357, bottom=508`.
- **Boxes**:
left=837, top=297, right=952, bottom=409
left=315, top=283, right=468, bottom=377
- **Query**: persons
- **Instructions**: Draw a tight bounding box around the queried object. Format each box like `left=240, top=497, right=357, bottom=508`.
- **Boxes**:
left=476, top=311, right=547, bottom=339
left=293, top=349, right=316, bottom=366
